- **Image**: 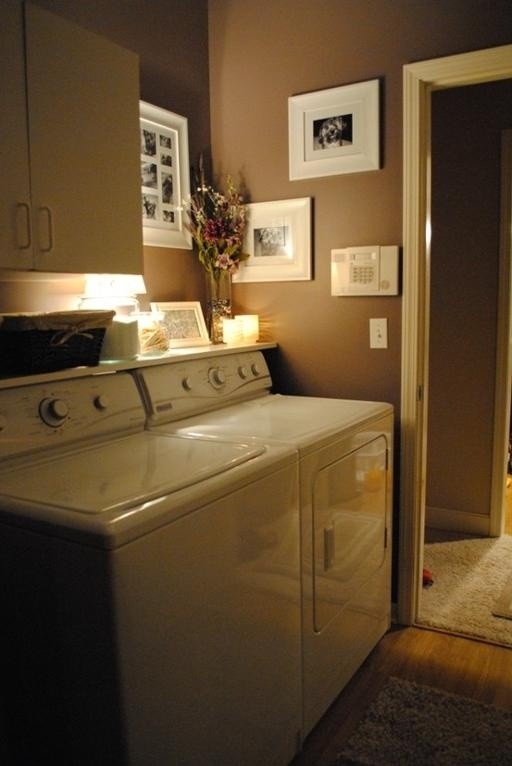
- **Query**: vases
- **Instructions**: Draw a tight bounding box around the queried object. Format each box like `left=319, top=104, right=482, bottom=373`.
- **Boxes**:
left=210, top=271, right=232, bottom=345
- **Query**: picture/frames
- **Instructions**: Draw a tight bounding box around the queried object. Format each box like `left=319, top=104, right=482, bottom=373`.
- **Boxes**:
left=139, top=99, right=193, bottom=250
left=151, top=302, right=210, bottom=349
left=288, top=78, right=380, bottom=181
left=232, top=197, right=312, bottom=283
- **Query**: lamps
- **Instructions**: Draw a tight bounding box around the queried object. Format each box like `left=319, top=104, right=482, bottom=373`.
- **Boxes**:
left=223, top=314, right=259, bottom=346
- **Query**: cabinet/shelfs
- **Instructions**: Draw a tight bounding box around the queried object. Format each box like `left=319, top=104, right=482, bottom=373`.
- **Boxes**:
left=0, top=0, right=144, bottom=275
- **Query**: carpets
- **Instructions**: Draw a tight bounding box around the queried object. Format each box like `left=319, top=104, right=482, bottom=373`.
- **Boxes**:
left=415, top=526, right=512, bottom=649
left=331, top=676, right=512, bottom=766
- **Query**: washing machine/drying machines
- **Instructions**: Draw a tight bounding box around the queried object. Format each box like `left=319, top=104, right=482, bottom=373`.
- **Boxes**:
left=1, top=360, right=300, bottom=765
left=134, top=354, right=394, bottom=749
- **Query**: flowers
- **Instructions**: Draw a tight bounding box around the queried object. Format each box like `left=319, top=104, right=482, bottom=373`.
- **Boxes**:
left=178, top=153, right=252, bottom=301
